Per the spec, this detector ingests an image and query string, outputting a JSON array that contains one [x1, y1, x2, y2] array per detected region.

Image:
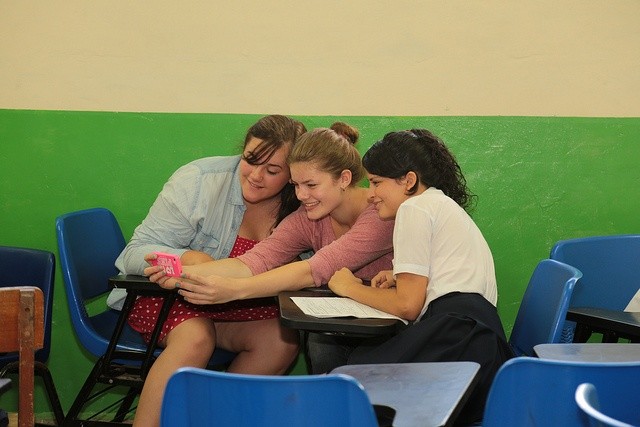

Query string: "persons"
[[103, 115, 309, 425], [142, 121, 393, 309], [326, 124, 514, 423]]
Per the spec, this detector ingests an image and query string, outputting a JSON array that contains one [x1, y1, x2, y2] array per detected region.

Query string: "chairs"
[[482, 357, 640, 427], [550, 235, 639, 342], [575, 383, 633, 426], [467, 258, 583, 427], [0, 286, 45, 427], [55, 207, 237, 427], [0, 246, 65, 426], [152, 366, 379, 427]]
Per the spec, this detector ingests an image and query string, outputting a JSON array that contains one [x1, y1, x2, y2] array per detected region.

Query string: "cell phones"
[[150, 252, 182, 280]]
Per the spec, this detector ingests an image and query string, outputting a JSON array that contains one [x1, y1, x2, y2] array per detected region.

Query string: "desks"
[[325, 361, 482, 427], [566, 307, 640, 343], [277, 289, 402, 337], [533, 343, 640, 364]]
